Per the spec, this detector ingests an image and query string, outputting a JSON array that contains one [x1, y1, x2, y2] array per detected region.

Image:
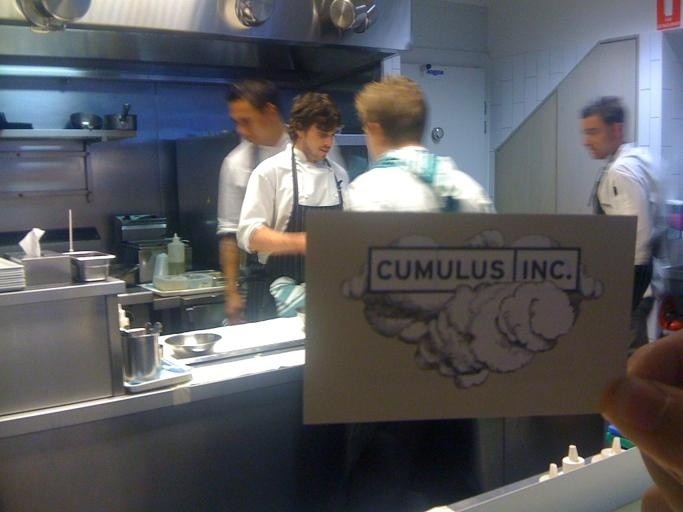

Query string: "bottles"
[[167, 233, 185, 275]]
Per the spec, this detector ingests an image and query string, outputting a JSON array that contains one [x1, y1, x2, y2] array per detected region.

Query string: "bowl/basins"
[[165, 333, 222, 357]]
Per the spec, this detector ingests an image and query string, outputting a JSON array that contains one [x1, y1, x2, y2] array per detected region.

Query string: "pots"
[[105, 103, 137, 130]]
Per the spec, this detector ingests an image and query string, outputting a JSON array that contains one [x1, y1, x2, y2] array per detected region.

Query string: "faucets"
[[120, 264, 140, 278]]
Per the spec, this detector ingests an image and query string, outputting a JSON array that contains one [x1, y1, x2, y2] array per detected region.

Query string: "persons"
[[334, 75, 496, 511], [596, 329, 682, 512], [577, 92, 666, 361], [214, 75, 292, 327], [236, 89, 349, 321]]
